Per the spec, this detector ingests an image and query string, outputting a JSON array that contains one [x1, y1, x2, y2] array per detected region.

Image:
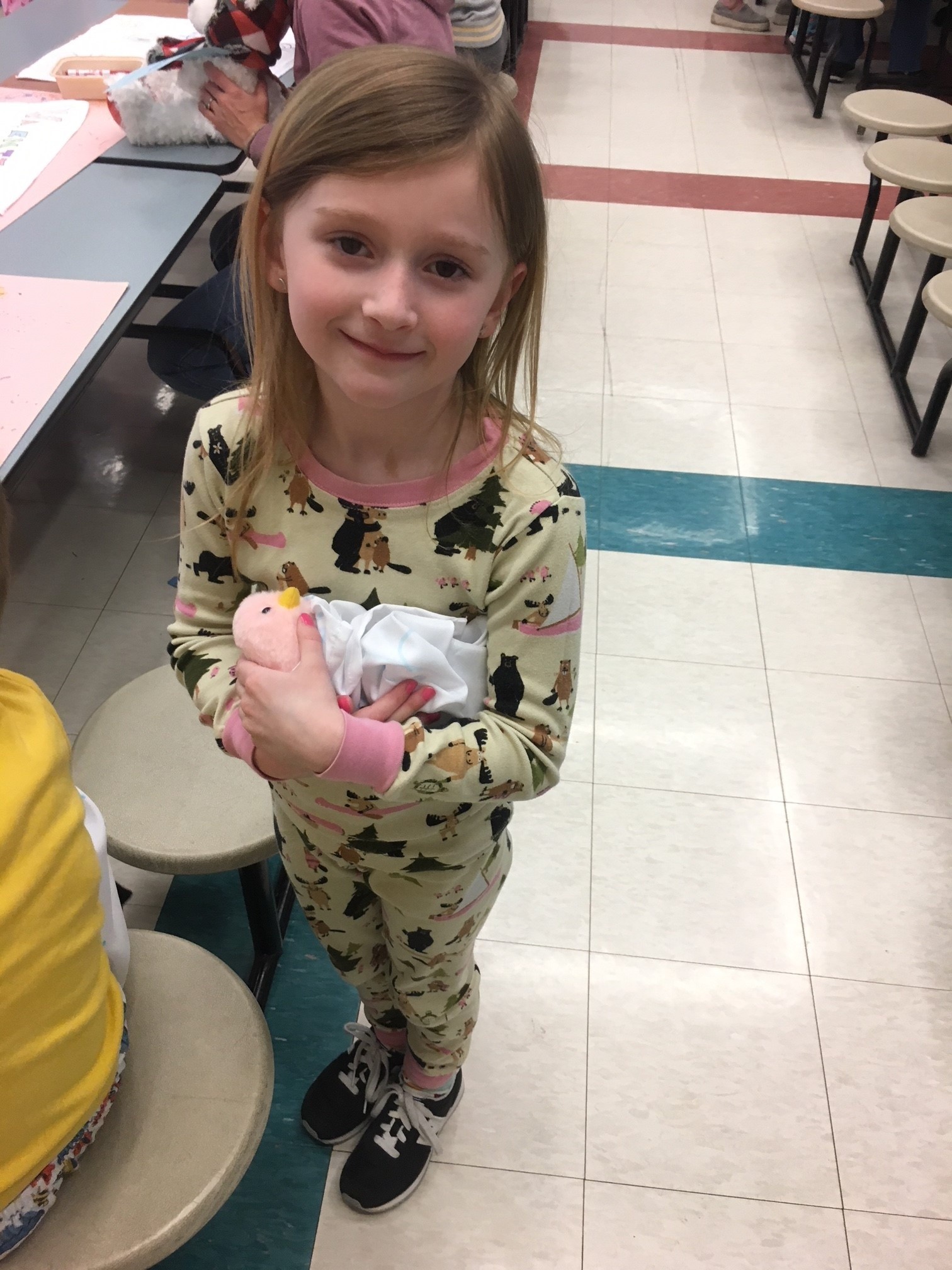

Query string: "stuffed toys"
[[106, 0, 296, 147], [232, 586, 486, 721]]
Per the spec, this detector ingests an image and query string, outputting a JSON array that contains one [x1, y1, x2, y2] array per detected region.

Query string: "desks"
[[0, 0, 248, 488]]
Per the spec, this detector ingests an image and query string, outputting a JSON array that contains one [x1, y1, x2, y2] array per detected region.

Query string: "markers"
[[64, 67, 133, 76]]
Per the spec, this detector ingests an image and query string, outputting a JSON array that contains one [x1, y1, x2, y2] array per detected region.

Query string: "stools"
[[785, 0, 952, 458], [0, 664, 302, 1270]]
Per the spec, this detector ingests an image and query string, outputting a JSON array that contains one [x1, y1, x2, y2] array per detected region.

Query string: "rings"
[[207, 97, 214, 109]]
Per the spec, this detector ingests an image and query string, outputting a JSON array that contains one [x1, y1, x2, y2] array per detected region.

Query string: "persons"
[[1, 670, 131, 1264], [165, 50, 587, 1213], [449, 0, 507, 79], [146, 0, 457, 400], [711, 0, 931, 90]]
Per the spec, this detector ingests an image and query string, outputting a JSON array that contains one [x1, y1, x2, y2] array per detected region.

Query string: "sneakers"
[[772, 0, 803, 26], [711, 0, 771, 32], [829, 60, 857, 84], [300, 1022, 404, 1145], [339, 1068, 465, 1214], [789, 22, 832, 57]]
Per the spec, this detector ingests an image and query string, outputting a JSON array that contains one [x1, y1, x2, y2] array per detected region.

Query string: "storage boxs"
[[51, 56, 144, 99]]
